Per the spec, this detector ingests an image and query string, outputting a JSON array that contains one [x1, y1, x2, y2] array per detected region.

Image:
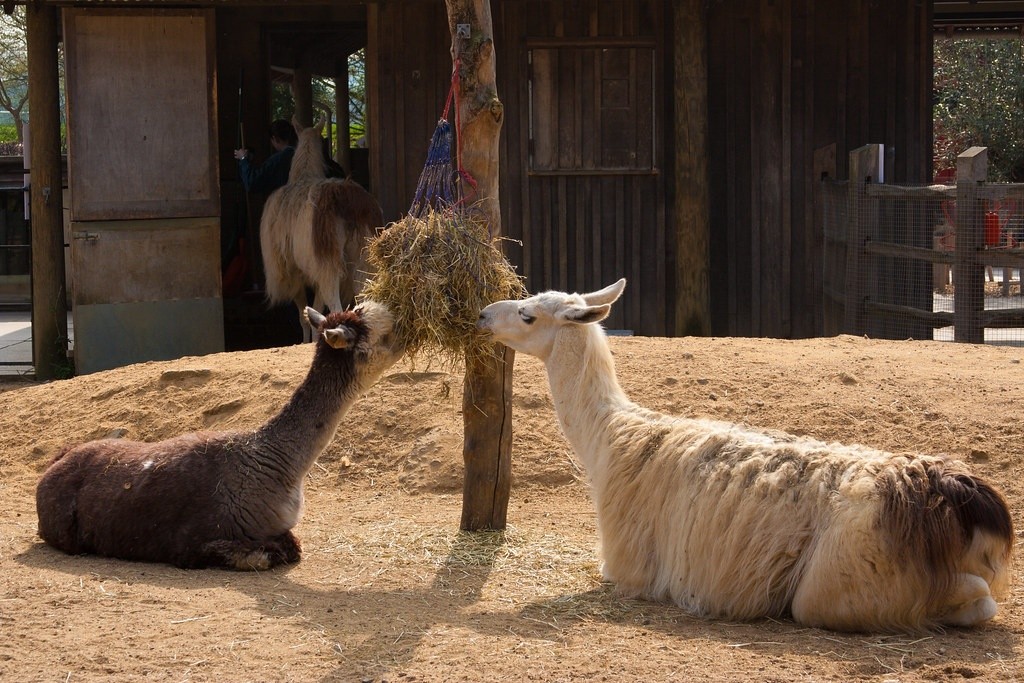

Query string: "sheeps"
[[259, 112, 387, 345], [479, 278, 1015, 639], [34, 299, 407, 575]]
